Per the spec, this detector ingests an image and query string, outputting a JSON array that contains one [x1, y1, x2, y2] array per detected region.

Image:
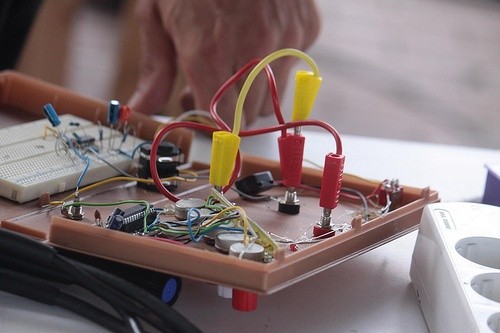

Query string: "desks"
[[0, 108, 500, 333]]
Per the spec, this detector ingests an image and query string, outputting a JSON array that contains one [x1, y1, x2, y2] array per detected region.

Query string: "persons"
[[0, 0, 322, 137]]
